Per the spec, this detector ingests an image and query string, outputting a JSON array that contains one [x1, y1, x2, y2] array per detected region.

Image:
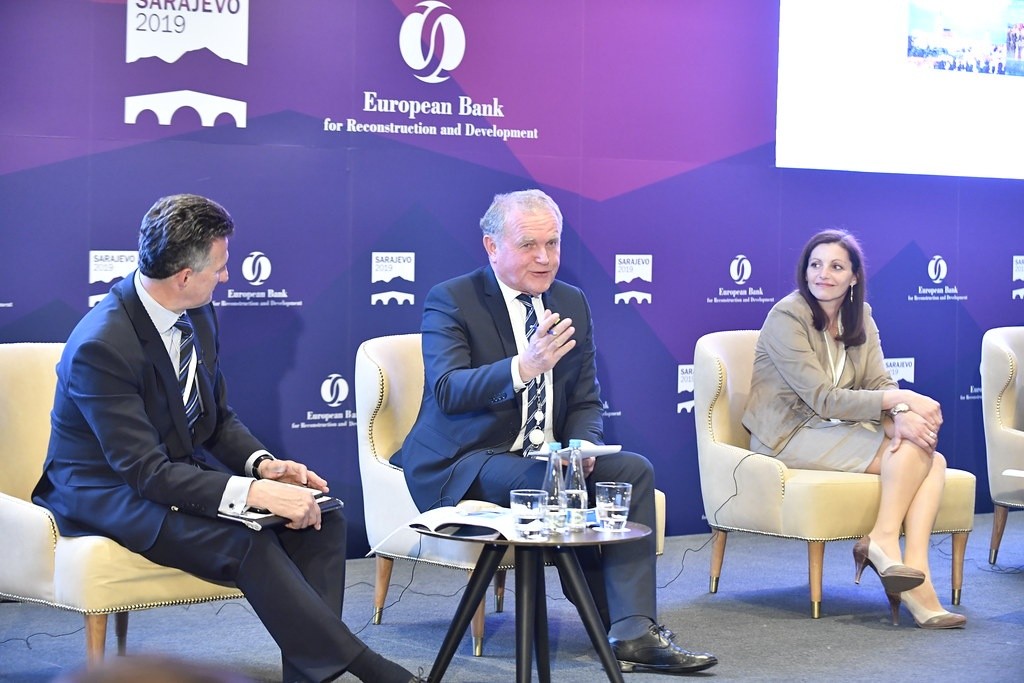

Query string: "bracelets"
[[251, 454, 274, 480]]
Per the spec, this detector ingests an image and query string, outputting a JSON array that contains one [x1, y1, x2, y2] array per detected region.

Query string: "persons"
[[30, 192, 429, 683], [388, 188, 718, 674], [741, 228, 968, 631]]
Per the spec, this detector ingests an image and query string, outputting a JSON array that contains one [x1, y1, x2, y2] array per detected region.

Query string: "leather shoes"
[[605, 623, 718, 672]]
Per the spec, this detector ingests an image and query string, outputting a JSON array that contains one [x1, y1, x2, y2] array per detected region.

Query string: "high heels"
[[852, 534, 926, 593], [885, 588, 967, 630]]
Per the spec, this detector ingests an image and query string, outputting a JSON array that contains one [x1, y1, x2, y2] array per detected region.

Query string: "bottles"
[[537, 441, 566, 505], [565, 439, 587, 491]]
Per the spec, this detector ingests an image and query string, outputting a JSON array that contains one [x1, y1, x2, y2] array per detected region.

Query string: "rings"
[[929, 432, 935, 438]]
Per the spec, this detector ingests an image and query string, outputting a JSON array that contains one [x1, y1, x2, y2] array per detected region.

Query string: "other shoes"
[[410, 667, 431, 683]]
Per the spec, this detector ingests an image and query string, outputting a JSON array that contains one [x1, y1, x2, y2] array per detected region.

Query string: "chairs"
[[354, 329, 666, 655], [978, 323, 1024, 567], [695, 328, 977, 620], [0, 343, 244, 683]]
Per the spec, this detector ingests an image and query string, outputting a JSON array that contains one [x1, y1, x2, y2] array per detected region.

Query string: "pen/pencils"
[[530, 325, 557, 336]]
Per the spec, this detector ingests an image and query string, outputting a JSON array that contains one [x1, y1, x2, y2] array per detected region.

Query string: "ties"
[[516, 292, 546, 459], [174, 314, 204, 472]]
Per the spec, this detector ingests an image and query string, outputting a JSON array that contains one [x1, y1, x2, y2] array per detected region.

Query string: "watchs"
[[890, 403, 911, 416]]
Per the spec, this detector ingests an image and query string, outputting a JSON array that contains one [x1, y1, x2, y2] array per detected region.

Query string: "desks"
[[400, 513, 657, 683]]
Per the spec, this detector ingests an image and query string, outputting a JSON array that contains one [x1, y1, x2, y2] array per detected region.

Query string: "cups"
[[558, 488, 588, 533], [510, 489, 549, 540], [540, 505, 565, 539], [596, 483, 632, 531]]
